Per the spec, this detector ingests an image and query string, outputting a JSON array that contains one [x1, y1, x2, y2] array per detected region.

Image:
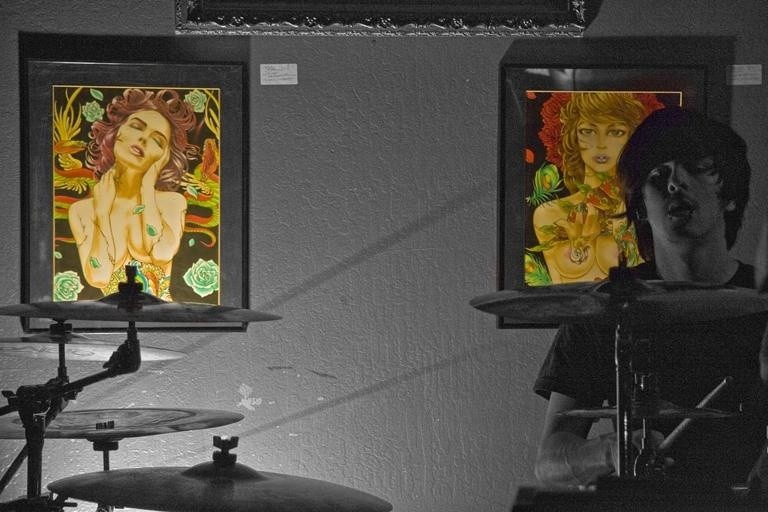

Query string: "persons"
[[68, 88, 200, 302], [532, 107, 768, 483], [533, 92, 646, 284]]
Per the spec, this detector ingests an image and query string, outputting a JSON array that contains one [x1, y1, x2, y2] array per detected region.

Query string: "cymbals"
[[48, 461, 392, 512], [469, 280, 767, 327], [0, 407, 244, 438], [0, 300, 283, 321], [557, 399, 738, 421], [0, 331, 188, 361]]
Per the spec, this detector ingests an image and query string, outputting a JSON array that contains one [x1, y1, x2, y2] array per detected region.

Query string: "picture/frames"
[[496, 60, 708, 331], [18, 56, 252, 333]]
